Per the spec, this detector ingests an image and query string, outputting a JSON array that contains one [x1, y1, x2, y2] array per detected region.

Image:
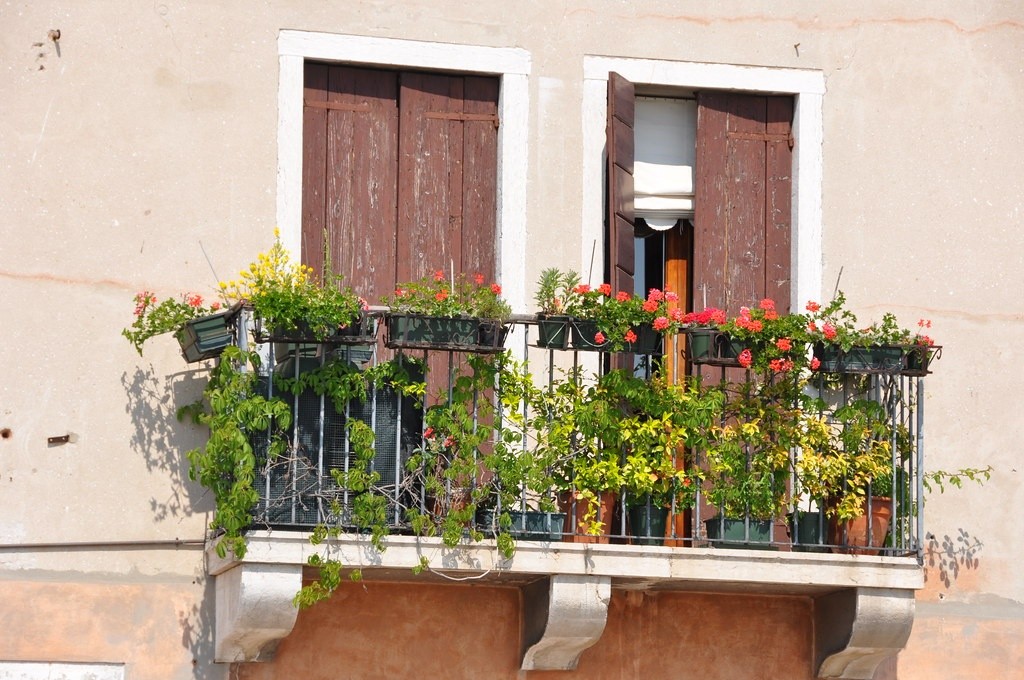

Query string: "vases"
[[786, 511, 831, 553], [272, 319, 337, 340], [702, 516, 771, 550], [902, 350, 933, 377], [475, 508, 568, 541], [536, 312, 658, 356], [338, 312, 361, 337], [274, 317, 376, 363], [628, 503, 671, 547], [389, 314, 480, 347], [687, 332, 765, 369], [173, 312, 233, 363], [481, 323, 509, 348], [827, 495, 893, 556], [811, 341, 904, 376]]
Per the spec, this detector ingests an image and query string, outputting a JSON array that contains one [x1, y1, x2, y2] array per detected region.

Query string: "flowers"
[[117, 223, 998, 616]]
